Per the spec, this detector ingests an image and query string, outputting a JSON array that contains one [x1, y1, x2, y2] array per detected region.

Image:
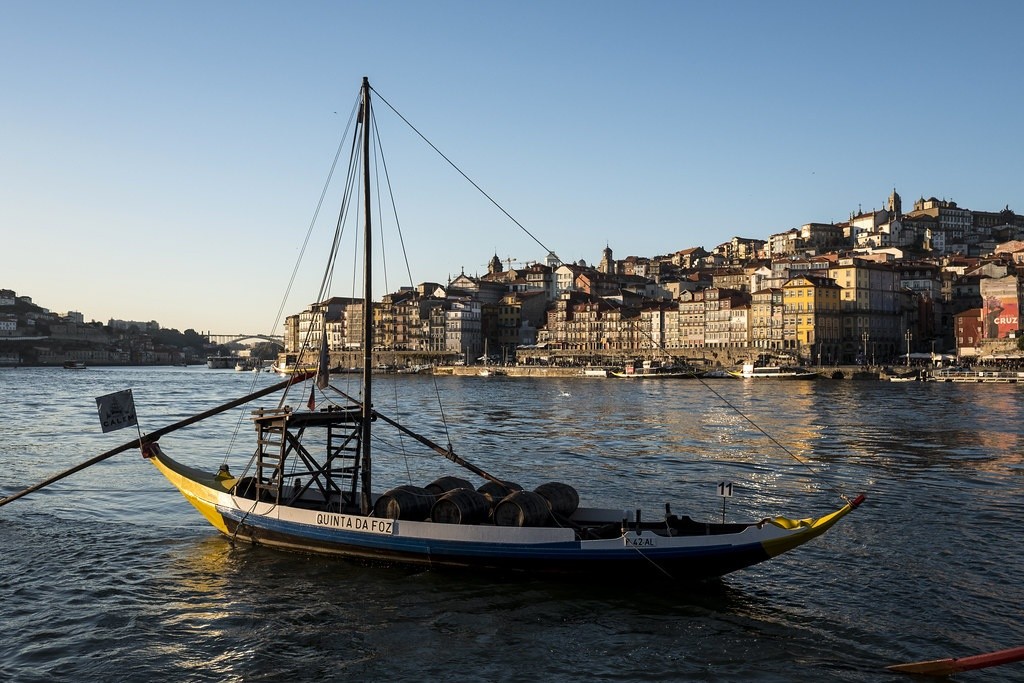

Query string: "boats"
[[889, 376, 918, 381], [608, 357, 705, 378], [725, 352, 826, 379]]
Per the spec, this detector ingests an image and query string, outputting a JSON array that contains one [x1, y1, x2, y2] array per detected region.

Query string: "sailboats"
[[97, 78, 872, 578], [475, 337, 496, 376]]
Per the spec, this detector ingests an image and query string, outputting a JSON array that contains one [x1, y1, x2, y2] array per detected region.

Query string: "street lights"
[[861, 331, 870, 363], [904, 329, 913, 366]]
